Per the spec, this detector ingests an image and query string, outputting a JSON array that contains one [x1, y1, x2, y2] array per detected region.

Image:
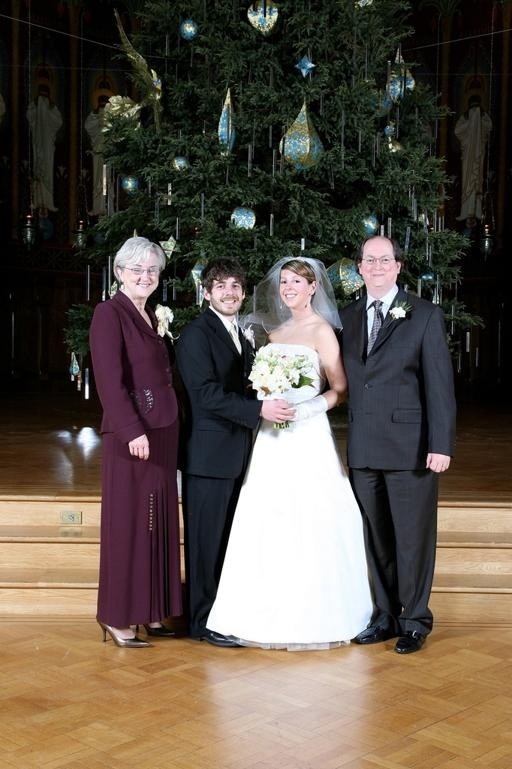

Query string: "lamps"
[[71, 186, 89, 251], [19, 177, 39, 252], [479, 191, 497, 262]]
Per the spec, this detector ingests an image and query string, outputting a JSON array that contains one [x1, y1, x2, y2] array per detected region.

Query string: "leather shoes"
[[190, 632, 242, 647], [395, 630, 427, 653], [355, 624, 394, 644]]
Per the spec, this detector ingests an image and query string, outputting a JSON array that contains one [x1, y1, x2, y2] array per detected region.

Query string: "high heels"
[[136, 622, 176, 637], [97, 619, 151, 648]]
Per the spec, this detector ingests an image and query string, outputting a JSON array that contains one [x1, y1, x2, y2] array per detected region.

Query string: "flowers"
[[155, 306, 181, 340], [389, 300, 413, 320], [244, 323, 256, 349], [247, 345, 316, 429]]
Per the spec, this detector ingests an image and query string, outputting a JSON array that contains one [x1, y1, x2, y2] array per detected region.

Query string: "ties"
[[231, 323, 244, 355], [367, 300, 384, 356]]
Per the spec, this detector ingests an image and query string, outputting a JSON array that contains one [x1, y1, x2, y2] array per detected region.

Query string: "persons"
[[88, 237, 183, 647], [172, 258, 296, 647], [205, 255, 374, 652], [330, 235, 458, 653]]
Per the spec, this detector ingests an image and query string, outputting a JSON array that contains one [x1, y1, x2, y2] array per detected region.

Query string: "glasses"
[[121, 266, 160, 275], [361, 256, 395, 265]]
